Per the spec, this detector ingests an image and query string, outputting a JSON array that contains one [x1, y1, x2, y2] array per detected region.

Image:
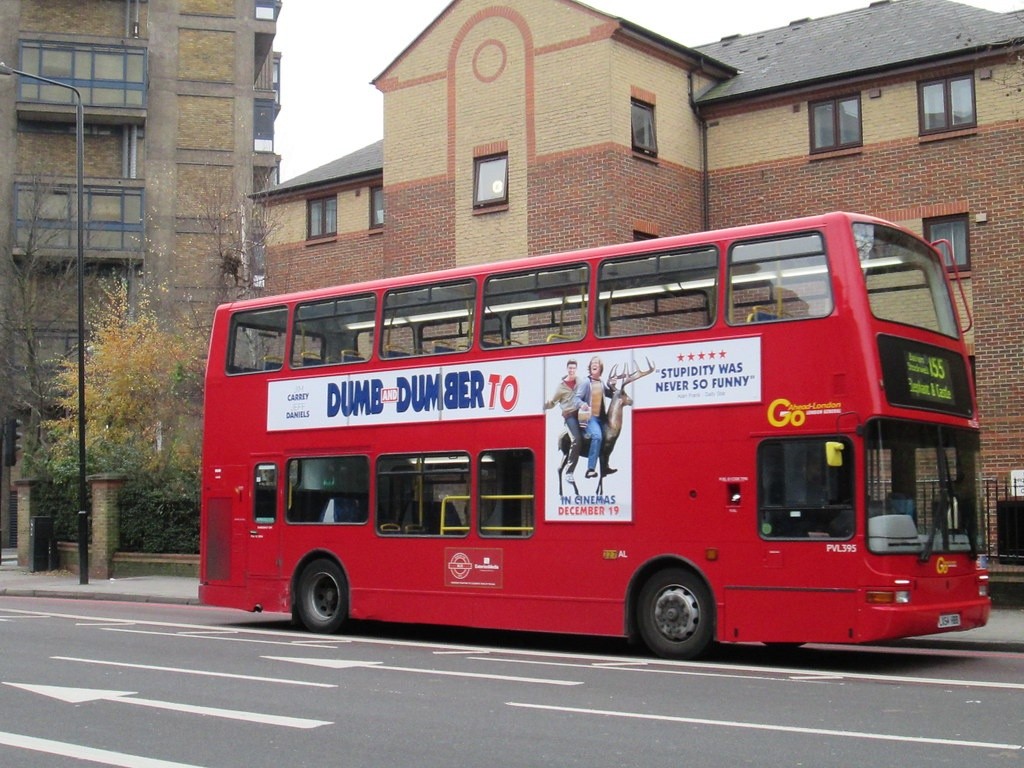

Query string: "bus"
[[198, 210, 992, 662]]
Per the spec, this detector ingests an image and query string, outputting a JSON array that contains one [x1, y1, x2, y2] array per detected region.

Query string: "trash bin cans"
[[29, 516, 52, 572]]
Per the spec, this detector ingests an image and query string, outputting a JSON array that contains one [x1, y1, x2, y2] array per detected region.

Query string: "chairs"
[[262, 334, 574, 370], [406, 524, 426, 534], [746, 307, 793, 323], [380, 524, 402, 534]]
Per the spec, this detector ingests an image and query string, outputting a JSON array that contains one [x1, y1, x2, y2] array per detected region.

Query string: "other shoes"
[[566, 474, 573, 482], [585, 470, 597, 478], [606, 467, 618, 475]]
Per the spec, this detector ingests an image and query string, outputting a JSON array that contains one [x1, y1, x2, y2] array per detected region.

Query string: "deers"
[[557, 356, 656, 498]]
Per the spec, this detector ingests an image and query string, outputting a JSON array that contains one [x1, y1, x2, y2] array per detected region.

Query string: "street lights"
[[0, 62, 88, 587]]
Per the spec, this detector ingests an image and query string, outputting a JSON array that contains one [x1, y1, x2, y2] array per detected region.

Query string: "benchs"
[[319, 497, 369, 523]]
